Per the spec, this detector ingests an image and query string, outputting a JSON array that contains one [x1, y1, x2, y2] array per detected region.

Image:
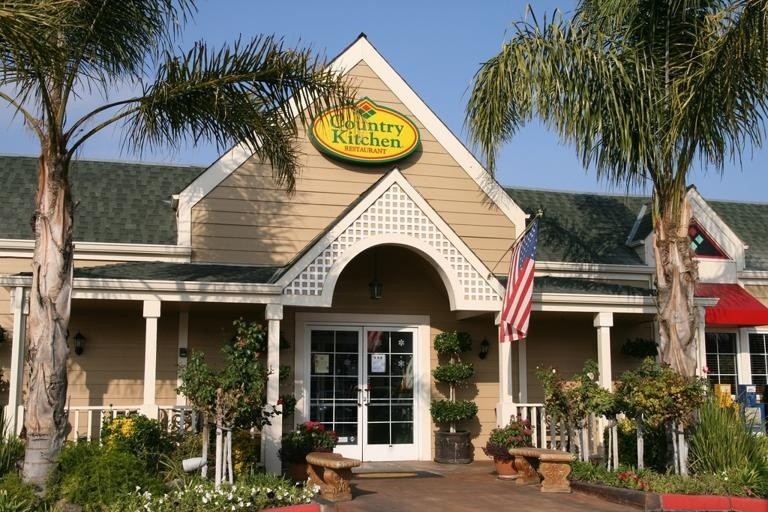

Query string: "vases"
[[281, 448, 333, 481], [493, 453, 519, 475]]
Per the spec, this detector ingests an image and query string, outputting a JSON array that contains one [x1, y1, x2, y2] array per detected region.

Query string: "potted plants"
[[430, 328, 478, 464]]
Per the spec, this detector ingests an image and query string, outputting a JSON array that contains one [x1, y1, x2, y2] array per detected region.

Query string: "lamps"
[[73, 330, 87, 356], [479, 337, 490, 359], [369, 246, 385, 300]]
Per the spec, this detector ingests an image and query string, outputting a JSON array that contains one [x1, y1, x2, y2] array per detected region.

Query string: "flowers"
[[281, 420, 338, 451], [481, 414, 536, 457]]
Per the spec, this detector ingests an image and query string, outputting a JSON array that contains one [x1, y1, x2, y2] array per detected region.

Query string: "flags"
[[498, 217, 538, 344]]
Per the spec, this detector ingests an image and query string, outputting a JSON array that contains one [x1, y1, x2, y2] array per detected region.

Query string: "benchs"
[[304, 450, 361, 501], [508, 447, 576, 494]]
